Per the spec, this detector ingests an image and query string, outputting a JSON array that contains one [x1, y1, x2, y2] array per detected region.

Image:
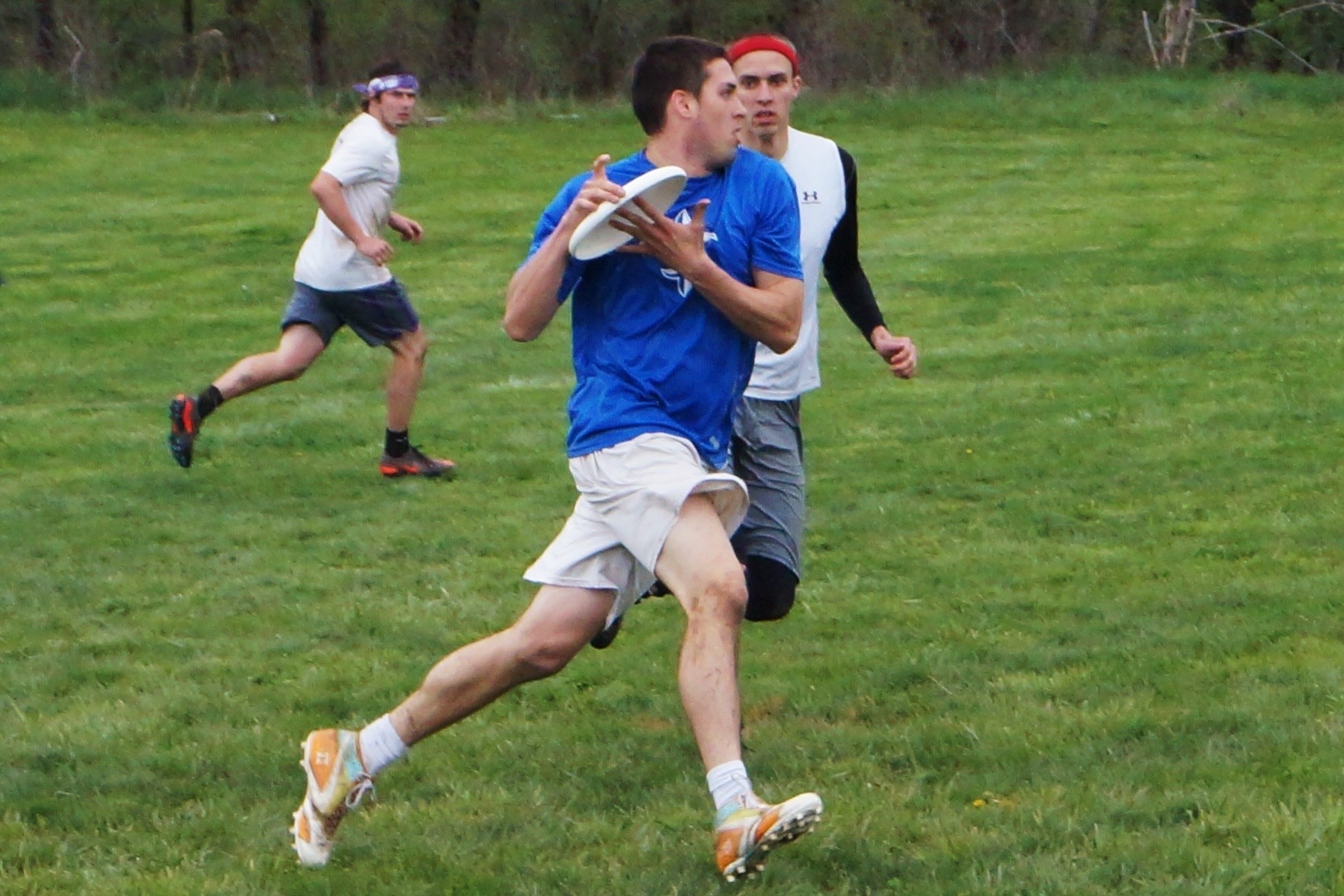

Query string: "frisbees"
[[567, 165, 689, 262]]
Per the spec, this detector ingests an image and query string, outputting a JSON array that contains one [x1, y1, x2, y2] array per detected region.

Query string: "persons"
[[287, 37, 825, 883], [168, 61, 455, 475], [590, 31, 917, 650]]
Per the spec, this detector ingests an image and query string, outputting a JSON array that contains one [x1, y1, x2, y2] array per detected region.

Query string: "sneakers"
[[590, 614, 623, 649], [294, 729, 364, 867], [382, 449, 453, 477], [717, 792, 824, 883], [169, 392, 199, 468]]
